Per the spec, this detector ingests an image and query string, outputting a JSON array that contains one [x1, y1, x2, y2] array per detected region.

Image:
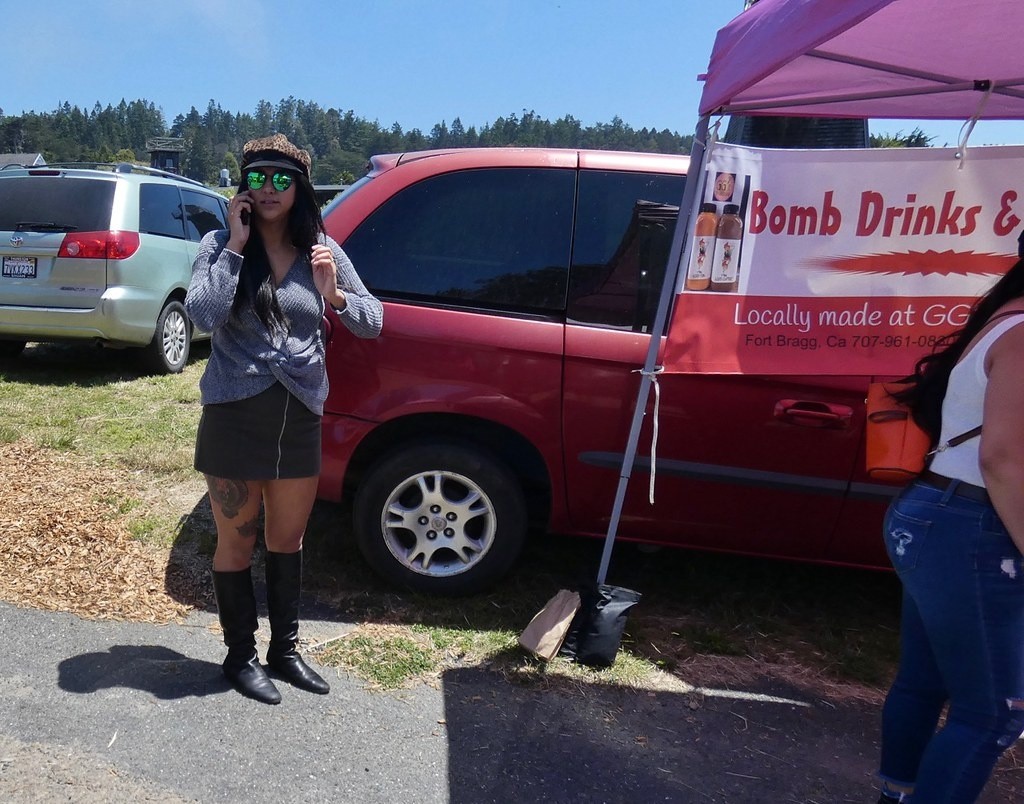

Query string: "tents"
[[592, 0, 1023, 593]]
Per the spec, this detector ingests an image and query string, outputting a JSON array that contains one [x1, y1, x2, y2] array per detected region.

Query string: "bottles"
[[686, 203, 720, 291], [711, 205, 743, 292]]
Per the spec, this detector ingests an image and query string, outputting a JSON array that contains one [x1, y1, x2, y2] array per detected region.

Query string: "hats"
[[243, 132, 311, 181]]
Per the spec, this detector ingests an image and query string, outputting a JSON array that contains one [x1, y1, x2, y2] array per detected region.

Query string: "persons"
[[184, 135, 384, 704], [877, 230, 1023, 804]]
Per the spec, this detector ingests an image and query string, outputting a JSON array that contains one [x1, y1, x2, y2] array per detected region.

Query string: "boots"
[[210, 565, 282, 704], [265, 549, 330, 694]]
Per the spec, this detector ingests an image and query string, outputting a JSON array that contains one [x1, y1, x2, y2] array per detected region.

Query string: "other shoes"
[[877, 792, 907, 804]]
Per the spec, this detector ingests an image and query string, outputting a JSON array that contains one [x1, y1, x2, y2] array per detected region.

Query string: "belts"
[[918, 469, 991, 502]]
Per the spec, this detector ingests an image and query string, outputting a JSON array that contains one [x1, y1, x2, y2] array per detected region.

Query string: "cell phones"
[[239, 184, 248, 224]]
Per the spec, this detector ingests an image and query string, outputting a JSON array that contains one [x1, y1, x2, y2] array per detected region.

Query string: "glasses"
[[246, 169, 296, 193]]
